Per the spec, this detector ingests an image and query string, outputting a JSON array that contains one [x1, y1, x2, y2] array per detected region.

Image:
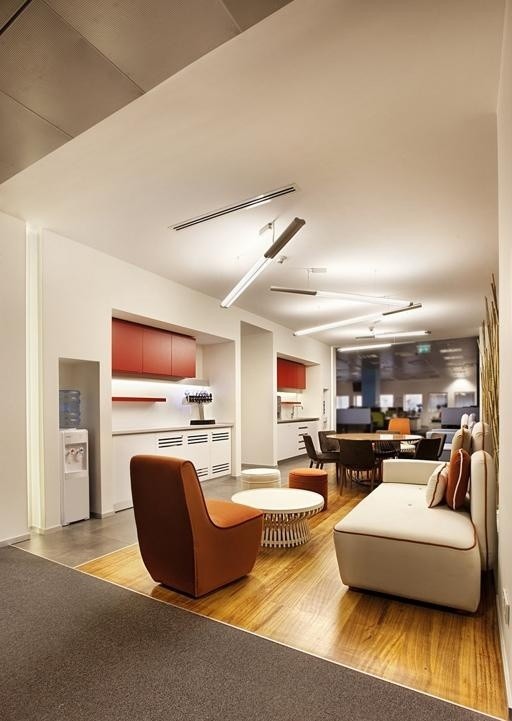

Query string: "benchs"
[[441, 407, 479, 427], [337, 408, 373, 432]]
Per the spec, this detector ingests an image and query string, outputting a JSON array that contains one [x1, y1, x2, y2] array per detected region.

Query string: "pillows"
[[424, 426, 471, 511]]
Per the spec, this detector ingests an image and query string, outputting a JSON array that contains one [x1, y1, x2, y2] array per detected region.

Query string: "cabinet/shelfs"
[[278, 422, 320, 460], [156, 427, 233, 483], [277, 358, 306, 390], [113, 318, 197, 380]]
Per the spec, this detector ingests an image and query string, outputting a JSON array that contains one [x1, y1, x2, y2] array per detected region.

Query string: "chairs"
[[303, 418, 447, 496], [130, 454, 264, 598]]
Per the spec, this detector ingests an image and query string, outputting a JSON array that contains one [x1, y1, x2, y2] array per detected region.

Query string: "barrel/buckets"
[[60, 389, 81, 428]]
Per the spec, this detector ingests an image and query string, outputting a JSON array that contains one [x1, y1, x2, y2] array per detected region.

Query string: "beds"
[[333, 460, 497, 614]]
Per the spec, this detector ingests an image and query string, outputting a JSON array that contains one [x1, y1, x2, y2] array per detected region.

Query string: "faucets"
[[292, 405, 303, 414]]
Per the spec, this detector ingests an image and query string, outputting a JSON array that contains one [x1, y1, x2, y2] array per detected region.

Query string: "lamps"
[[219, 217, 432, 354]]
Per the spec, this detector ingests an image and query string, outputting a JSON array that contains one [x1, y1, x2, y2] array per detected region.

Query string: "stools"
[[241, 468, 281, 488], [289, 468, 328, 511]]
